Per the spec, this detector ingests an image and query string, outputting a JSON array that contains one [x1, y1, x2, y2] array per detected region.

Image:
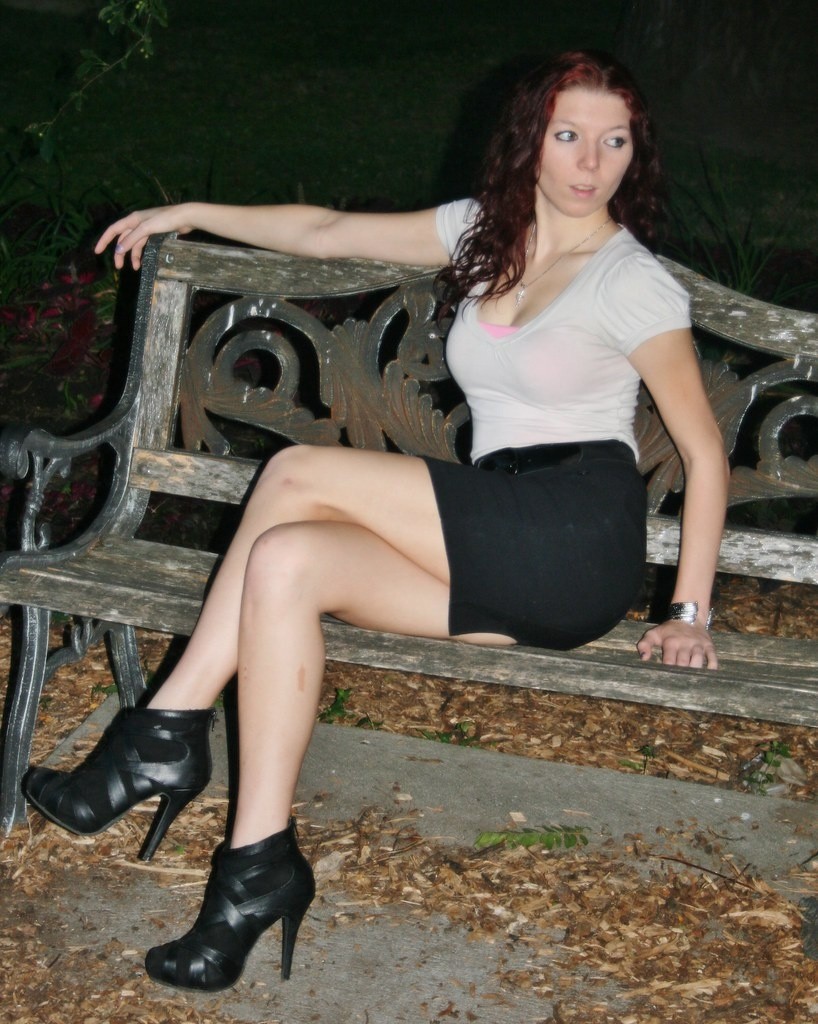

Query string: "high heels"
[[23, 706, 217, 862], [144, 817, 315, 993]]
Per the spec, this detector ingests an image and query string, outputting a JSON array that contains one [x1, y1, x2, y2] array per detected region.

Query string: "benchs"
[[0, 233, 817, 855]]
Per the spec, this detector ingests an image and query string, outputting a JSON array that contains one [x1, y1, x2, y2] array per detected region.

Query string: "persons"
[[19, 43, 731, 993]]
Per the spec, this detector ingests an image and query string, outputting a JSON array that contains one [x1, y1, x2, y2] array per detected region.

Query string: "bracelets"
[[665, 602, 714, 630]]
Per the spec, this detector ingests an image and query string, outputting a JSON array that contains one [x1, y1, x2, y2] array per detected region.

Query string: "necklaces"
[[515, 217, 613, 306]]
